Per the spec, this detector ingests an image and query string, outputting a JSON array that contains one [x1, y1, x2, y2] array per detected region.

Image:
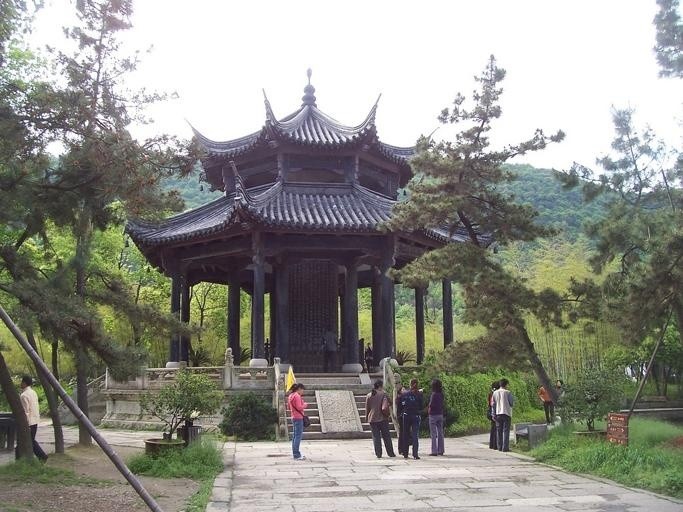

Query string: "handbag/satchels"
[[300, 415, 312, 428], [382, 397, 391, 416], [424, 415, 447, 426], [486, 410, 496, 424]]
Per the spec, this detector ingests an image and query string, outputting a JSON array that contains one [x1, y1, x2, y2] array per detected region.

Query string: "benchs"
[[510, 421, 550, 451]]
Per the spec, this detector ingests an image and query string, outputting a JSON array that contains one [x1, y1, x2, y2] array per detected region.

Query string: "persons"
[[538, 386, 556, 427], [364, 379, 396, 457], [287, 382, 306, 461], [320, 324, 373, 373], [488, 381, 500, 451], [491, 377, 514, 452], [555, 379, 567, 403], [398, 378, 424, 459], [14, 375, 48, 464], [394, 386, 413, 455], [428, 378, 446, 457]]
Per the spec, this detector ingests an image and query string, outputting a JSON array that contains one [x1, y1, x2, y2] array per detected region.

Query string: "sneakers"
[[293, 455, 307, 462], [375, 451, 446, 460], [489, 445, 513, 454]]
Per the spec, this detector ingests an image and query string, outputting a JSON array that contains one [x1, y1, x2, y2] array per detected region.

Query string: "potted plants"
[[566, 366, 636, 439], [137, 368, 229, 465]]
